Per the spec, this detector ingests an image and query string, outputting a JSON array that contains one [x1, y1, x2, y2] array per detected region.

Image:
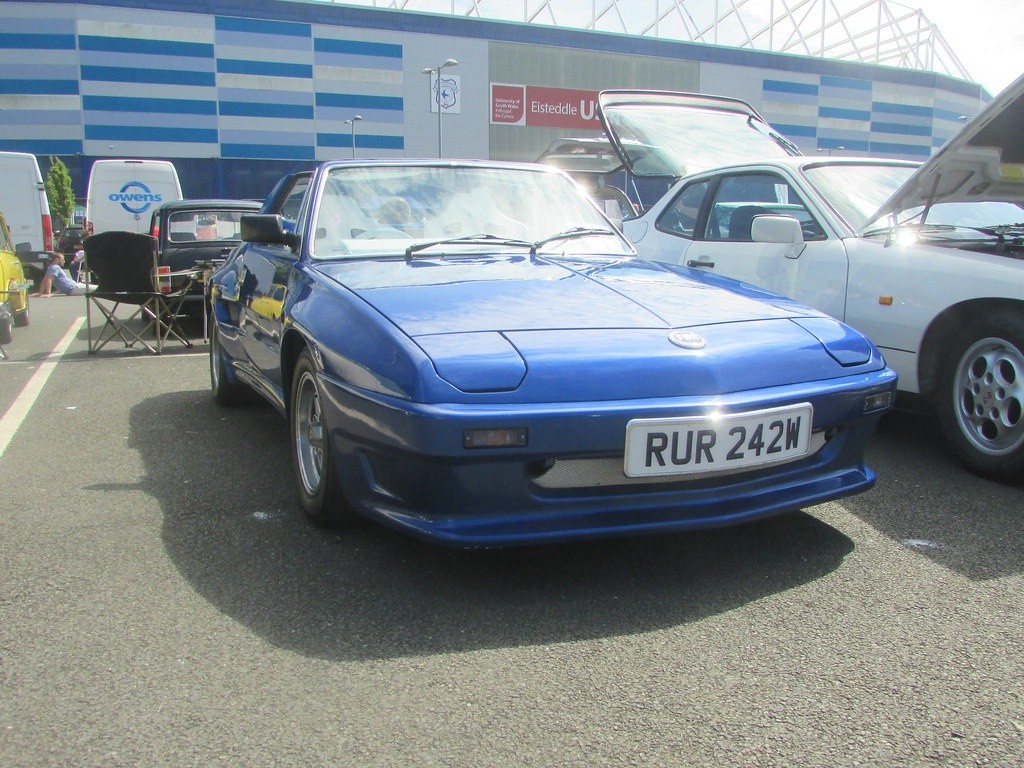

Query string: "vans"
[[0, 151, 53, 291], [83, 160, 184, 236]]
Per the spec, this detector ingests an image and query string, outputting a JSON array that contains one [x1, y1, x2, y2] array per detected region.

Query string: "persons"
[[33, 252, 98, 301], [671, 177, 709, 226], [69, 250, 85, 282], [376, 197, 423, 238], [623, 204, 639, 220], [195, 219, 218, 240]]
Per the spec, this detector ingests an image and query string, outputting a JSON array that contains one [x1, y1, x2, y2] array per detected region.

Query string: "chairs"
[[172, 231, 197, 241], [83, 231, 199, 355], [728, 205, 772, 240]]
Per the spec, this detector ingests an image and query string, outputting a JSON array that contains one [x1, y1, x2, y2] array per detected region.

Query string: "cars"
[[0, 212, 30, 344], [532, 138, 662, 237], [54, 224, 86, 259], [589, 71, 1024, 478], [207, 159, 899, 551]]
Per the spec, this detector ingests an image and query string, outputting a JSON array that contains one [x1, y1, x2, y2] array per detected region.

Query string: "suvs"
[[139, 198, 294, 322]]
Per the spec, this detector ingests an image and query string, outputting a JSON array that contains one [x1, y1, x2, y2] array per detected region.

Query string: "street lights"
[[421, 59, 459, 159], [344, 115, 362, 159]]
